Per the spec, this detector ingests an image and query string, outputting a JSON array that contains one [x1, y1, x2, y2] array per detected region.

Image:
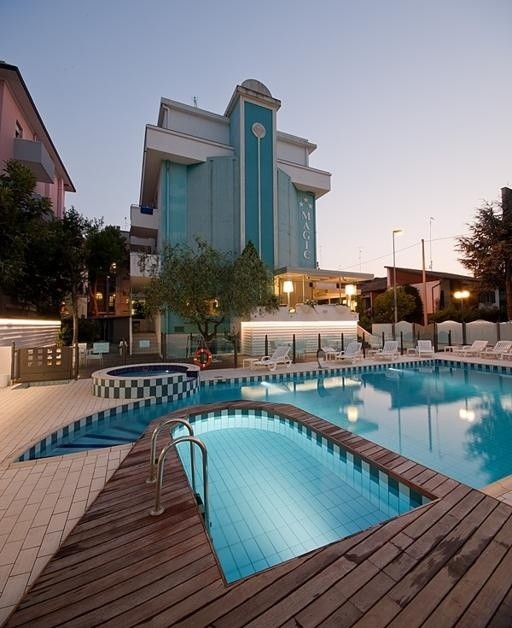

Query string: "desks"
[[324, 352, 339, 362], [243, 358, 259, 369]]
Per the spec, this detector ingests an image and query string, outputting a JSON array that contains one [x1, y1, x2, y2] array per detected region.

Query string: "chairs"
[[252, 345, 293, 372], [334, 339, 512, 364]]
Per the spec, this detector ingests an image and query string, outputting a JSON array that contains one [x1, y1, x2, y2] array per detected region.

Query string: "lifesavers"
[[193, 349, 212, 368]]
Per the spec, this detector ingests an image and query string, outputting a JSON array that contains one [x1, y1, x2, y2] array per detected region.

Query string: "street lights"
[[281, 278, 294, 306], [344, 387, 359, 425], [344, 282, 359, 309], [456, 396, 475, 422], [453, 289, 473, 322], [392, 227, 405, 321]]
